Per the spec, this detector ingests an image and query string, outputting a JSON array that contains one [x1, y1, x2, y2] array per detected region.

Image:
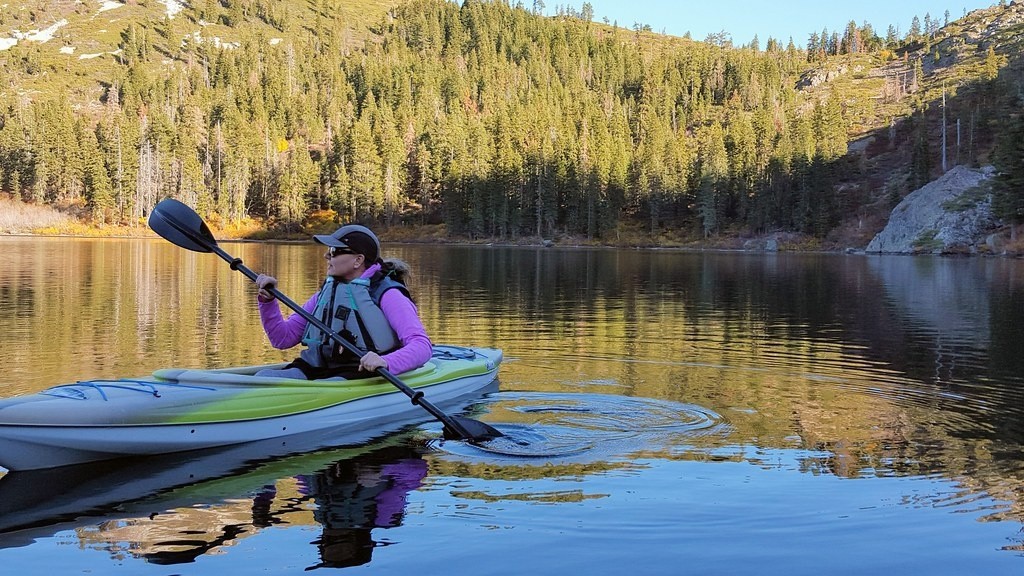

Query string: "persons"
[[251, 446, 429, 571], [255, 224, 433, 382]]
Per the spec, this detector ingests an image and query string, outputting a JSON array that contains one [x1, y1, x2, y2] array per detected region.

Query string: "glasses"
[[328, 246, 359, 258]]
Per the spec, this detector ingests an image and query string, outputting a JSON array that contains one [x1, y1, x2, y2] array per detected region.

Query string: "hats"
[[312, 224, 380, 263]]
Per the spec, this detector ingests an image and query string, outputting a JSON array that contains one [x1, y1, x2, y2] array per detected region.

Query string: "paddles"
[[149, 197, 499, 444]]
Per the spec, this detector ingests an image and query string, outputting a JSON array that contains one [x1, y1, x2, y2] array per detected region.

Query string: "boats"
[[0, 340, 507, 472]]
